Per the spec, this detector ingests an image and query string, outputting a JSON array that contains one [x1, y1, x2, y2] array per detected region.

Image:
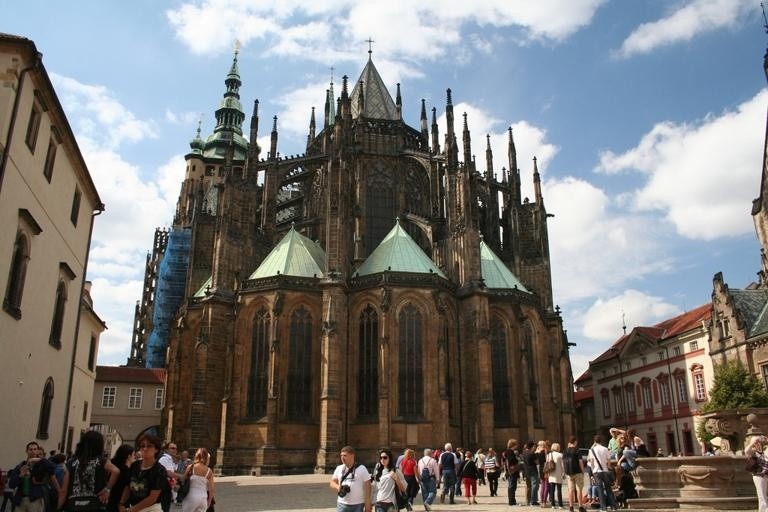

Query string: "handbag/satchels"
[[161, 480, 171, 512], [397, 493, 408, 509], [177, 475, 189, 502], [745, 455, 768, 475], [543, 460, 555, 473], [598, 472, 615, 483]]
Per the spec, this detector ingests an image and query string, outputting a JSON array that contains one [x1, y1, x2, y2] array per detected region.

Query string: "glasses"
[[380, 456, 387, 459]]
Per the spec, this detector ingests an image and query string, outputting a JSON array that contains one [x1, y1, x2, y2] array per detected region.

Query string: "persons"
[[678, 450, 684, 458], [701, 449, 713, 456], [654, 448, 666, 459], [667, 452, 675, 458], [330, 426, 650, 511], [745, 435, 768, 512], [0, 427, 217, 512]]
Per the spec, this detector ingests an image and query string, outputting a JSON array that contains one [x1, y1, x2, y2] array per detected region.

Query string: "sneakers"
[[424, 502, 431, 511], [407, 502, 412, 511], [449, 501, 456, 504], [600, 506, 616, 512], [441, 493, 445, 502], [491, 490, 497, 496], [527, 500, 595, 512], [468, 501, 477, 504]]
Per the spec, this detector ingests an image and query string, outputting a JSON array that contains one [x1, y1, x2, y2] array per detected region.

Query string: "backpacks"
[[564, 448, 580, 475], [421, 458, 432, 482]]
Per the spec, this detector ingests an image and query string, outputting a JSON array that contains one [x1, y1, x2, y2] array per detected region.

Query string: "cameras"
[[338, 486, 350, 498]]
